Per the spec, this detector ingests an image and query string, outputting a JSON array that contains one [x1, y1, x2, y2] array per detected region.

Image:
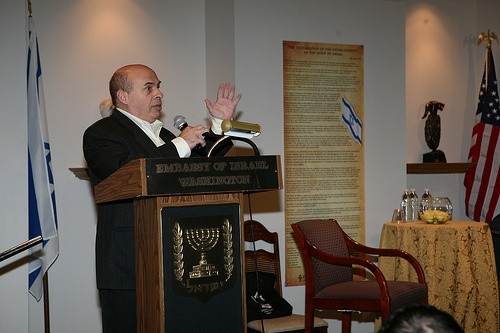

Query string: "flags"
[[26, 14, 60, 301], [463, 47, 500, 224]]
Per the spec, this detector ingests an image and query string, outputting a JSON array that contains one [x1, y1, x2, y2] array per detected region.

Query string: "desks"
[[378, 221, 499, 333]]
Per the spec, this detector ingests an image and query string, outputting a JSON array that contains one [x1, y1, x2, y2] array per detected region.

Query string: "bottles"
[[410, 189, 419, 222], [401, 189, 412, 222], [422, 188, 432, 199]]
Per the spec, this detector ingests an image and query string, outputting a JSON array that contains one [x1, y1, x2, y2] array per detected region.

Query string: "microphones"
[[173, 115, 207, 157]]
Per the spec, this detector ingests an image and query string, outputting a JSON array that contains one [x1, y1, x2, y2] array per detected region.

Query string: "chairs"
[[290, 218, 428, 333], [245, 221, 329, 333]]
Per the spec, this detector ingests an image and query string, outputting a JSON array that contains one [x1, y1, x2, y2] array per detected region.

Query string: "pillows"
[[246, 272, 294, 322]]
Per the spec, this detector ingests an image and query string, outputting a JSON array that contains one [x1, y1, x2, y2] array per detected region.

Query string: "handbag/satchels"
[[247, 272, 293, 323]]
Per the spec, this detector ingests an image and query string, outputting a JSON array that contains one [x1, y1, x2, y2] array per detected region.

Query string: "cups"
[[392, 208, 403, 224], [419, 196, 452, 223]]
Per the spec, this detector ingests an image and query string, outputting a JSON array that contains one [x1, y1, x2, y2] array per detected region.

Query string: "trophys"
[[186, 228, 221, 278]]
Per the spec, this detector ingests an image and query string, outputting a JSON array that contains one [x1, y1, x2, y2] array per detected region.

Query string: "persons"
[[83, 65, 242, 333]]
